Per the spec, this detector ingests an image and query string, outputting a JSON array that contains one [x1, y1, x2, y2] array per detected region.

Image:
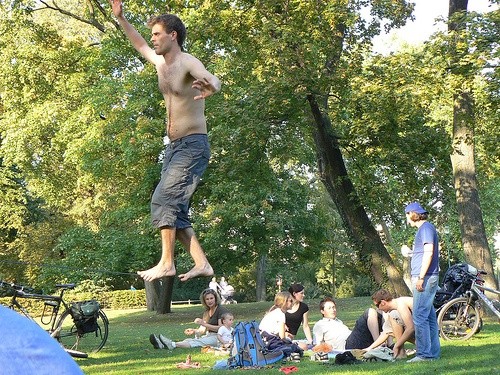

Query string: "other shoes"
[[407, 357, 426, 362], [404, 348, 416, 355]]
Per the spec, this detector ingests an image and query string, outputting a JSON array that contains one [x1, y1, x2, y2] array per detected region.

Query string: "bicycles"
[[436, 269, 500, 343], [0, 280, 110, 357]]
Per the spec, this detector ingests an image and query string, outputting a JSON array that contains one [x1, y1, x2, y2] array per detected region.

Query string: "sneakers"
[[309, 352, 329, 363], [150, 333, 163, 350], [159, 334, 174, 350], [343, 351, 362, 363], [335, 354, 362, 364], [282, 352, 300, 364]]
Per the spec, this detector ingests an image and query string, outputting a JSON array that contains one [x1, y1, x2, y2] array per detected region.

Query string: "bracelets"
[[418, 277, 424, 280]]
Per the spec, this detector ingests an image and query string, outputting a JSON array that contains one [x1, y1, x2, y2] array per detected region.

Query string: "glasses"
[[287, 299, 293, 303], [375, 299, 384, 308]]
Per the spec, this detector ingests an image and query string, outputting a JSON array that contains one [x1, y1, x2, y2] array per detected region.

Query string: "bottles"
[[194, 330, 200, 339], [186, 355, 190, 365]]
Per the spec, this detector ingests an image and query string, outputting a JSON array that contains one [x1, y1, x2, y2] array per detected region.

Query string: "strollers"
[[432, 263, 488, 320], [217, 288, 237, 305]]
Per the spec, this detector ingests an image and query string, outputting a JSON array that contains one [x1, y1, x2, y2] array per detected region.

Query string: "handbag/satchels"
[[71, 301, 99, 334]]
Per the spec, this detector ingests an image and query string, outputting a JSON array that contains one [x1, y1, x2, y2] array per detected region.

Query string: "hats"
[[405, 203, 427, 214]]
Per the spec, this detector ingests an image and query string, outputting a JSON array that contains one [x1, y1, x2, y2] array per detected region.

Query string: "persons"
[[271, 284, 312, 346], [0, 305, 86, 375], [362, 289, 416, 359], [209, 277, 223, 301], [150, 288, 228, 350], [217, 312, 234, 347], [107, 0, 221, 281], [312, 299, 396, 353], [401, 203, 442, 362], [219, 276, 227, 290], [258, 292, 293, 344]]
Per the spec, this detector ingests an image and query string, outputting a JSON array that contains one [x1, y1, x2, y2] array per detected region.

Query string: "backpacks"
[[227, 320, 266, 370]]
